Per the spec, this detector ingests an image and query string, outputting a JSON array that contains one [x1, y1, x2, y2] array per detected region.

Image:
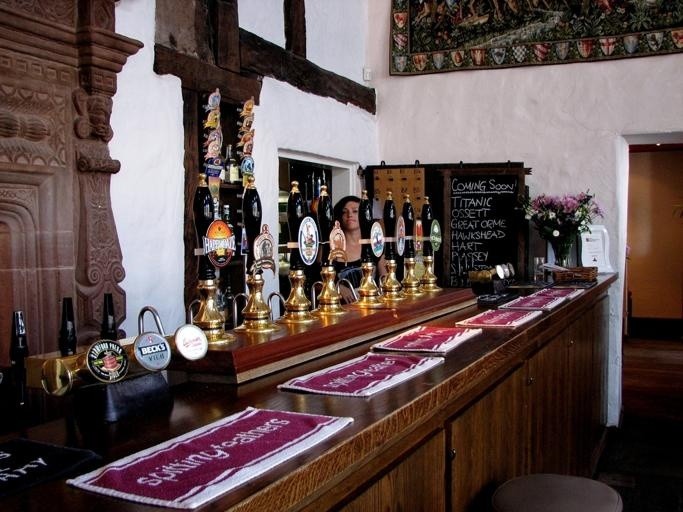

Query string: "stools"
[[489, 473, 623, 511]]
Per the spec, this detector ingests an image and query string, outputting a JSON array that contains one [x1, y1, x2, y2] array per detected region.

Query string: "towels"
[[497, 288, 583, 309], [371, 325, 482, 353], [66, 403, 356, 509], [454, 309, 545, 330], [274, 351, 447, 397]]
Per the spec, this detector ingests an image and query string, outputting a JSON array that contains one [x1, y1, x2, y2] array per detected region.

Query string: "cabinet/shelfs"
[[184, 82, 250, 332]]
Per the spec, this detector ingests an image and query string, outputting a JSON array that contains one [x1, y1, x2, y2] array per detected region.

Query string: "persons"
[[329, 195, 364, 305]]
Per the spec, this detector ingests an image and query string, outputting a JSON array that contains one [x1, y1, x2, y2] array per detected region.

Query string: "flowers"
[[515, 186, 605, 267]]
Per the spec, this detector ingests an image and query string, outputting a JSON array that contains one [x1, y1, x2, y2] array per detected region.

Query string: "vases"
[[552, 243, 574, 270]]
